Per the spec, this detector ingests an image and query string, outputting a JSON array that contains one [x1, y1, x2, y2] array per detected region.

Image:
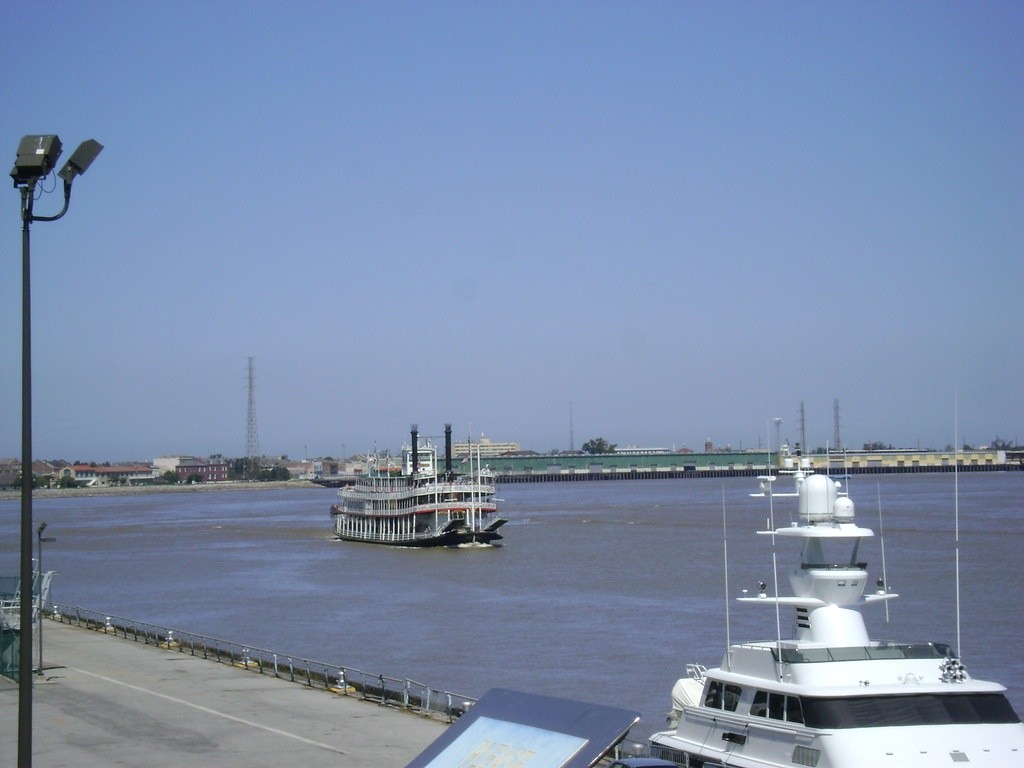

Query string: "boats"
[[638, 418, 1022, 768], [327, 423, 509, 544]]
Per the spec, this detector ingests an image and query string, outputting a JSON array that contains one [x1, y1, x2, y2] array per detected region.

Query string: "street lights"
[[32, 523, 49, 677], [7, 134, 104, 754]]
